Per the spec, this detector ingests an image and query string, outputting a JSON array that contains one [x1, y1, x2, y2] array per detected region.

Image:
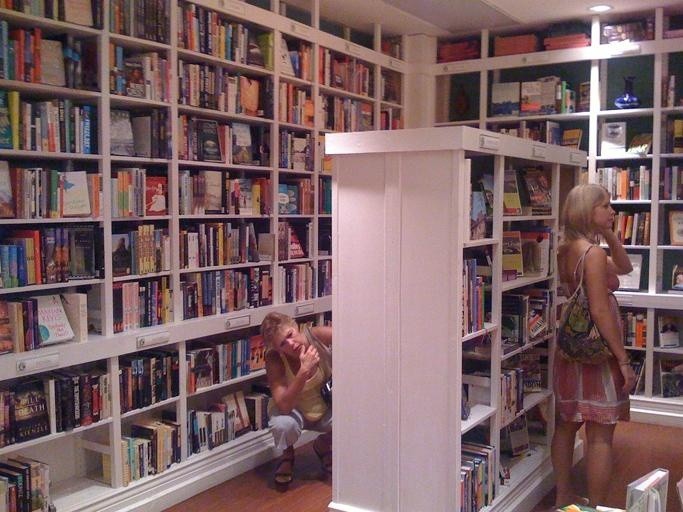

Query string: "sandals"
[[274, 457, 296, 486], [313, 434, 332, 474]]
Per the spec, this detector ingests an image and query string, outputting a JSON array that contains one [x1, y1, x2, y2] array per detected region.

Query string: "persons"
[[548, 181, 639, 508], [260, 310, 333, 485]]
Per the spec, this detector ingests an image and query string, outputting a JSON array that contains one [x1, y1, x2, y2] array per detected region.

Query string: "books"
[[459, 162, 557, 512], [278, 14, 408, 326], [85, 0, 180, 488], [176, 0, 271, 456], [1, 0, 112, 512], [437, 13, 683, 398]]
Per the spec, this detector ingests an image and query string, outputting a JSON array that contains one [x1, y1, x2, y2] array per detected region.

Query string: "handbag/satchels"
[[555, 277, 625, 367]]
